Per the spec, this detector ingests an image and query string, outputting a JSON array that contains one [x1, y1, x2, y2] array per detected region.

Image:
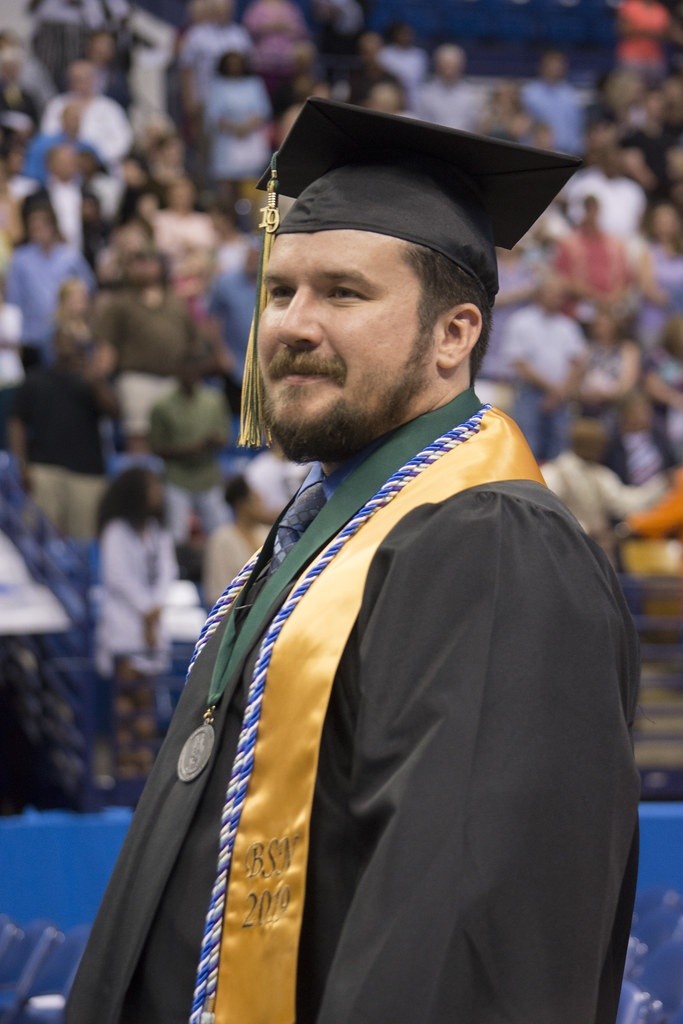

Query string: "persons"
[[66, 98, 649, 1024], [2, 0, 680, 787]]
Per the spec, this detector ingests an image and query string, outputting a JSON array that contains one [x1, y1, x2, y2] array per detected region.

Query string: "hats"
[[238, 93, 591, 446]]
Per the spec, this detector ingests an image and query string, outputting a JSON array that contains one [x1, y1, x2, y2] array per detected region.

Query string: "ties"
[[265, 482, 330, 583]]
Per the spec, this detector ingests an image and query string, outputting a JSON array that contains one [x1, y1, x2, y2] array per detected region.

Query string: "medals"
[[176, 718, 217, 784]]
